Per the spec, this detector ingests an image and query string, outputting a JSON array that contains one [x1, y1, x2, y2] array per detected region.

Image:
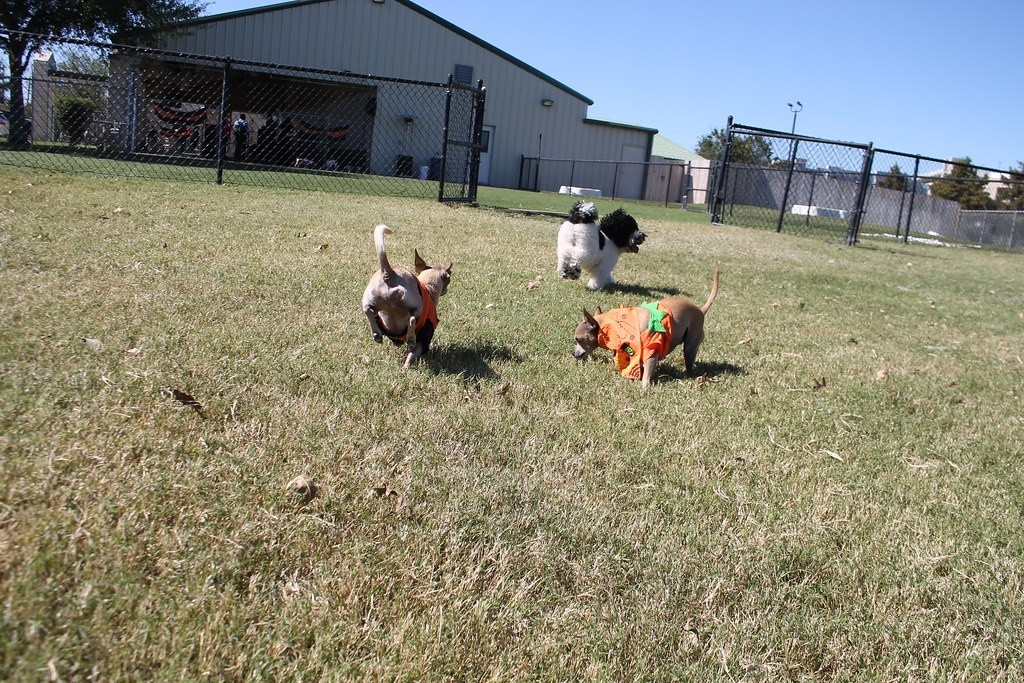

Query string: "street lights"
[[787, 101, 802, 168]]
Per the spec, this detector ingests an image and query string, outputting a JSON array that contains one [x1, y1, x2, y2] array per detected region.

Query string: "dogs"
[[571, 268, 719, 384], [362, 224, 455, 367], [555, 201, 650, 290]]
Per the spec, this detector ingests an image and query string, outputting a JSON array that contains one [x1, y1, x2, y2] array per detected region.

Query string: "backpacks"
[[232, 121, 246, 138]]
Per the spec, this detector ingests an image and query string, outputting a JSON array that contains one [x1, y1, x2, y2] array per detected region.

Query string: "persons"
[[233, 114, 249, 162]]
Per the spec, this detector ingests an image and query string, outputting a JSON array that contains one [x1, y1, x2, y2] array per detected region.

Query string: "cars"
[[4, 111, 31, 135], [0, 111, 10, 137]]
